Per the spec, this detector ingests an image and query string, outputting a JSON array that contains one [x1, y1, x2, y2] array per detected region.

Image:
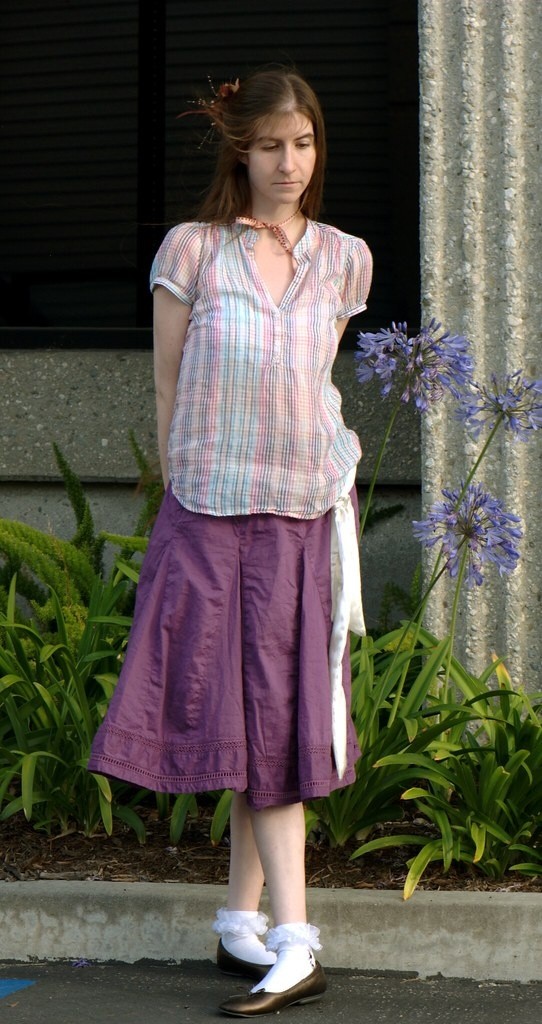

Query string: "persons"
[[87, 69, 373, 1018]]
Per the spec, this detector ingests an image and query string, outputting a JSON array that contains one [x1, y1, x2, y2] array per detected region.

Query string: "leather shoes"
[[218, 960, 329, 1018], [217, 937, 275, 974]]
